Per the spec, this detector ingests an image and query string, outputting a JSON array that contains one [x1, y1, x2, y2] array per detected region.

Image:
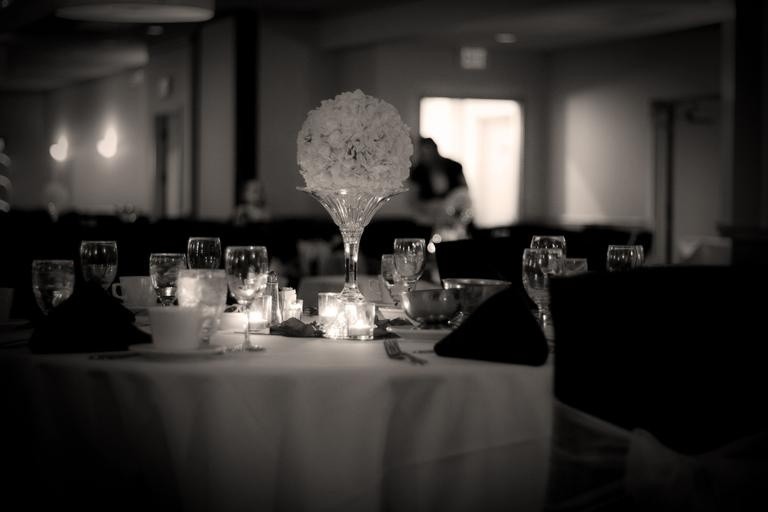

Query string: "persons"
[[405, 138, 474, 227], [234, 181, 273, 225]]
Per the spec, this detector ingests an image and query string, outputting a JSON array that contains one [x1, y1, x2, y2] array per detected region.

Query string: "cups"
[[31, 259, 75, 316], [111, 276, 158, 305], [317, 292, 342, 328], [606, 244, 645, 272], [145, 237, 228, 349], [0, 288, 14, 322], [220, 312, 249, 333], [75, 240, 118, 291], [242, 296, 272, 332], [343, 301, 377, 341]]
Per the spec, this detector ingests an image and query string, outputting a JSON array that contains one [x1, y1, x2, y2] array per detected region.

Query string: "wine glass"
[[224, 246, 269, 354], [393, 237, 427, 292], [521, 235, 588, 354], [381, 254, 401, 303]]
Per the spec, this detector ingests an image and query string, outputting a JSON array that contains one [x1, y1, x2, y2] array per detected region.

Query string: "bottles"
[[265, 270, 283, 327]]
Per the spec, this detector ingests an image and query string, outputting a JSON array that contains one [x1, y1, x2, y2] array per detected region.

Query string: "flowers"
[[296, 89, 415, 209]]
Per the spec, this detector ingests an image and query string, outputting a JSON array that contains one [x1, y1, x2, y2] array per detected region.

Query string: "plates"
[[129, 341, 228, 359], [0, 319, 30, 330], [121, 305, 151, 318], [378, 307, 405, 322]]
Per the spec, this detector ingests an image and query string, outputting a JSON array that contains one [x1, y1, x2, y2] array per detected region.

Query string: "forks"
[[383, 339, 429, 366]]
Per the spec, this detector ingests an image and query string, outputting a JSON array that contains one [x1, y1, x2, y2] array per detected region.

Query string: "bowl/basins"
[[399, 288, 462, 329], [442, 278, 512, 317]]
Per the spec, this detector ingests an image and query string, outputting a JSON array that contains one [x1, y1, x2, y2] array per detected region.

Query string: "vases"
[[294, 185, 410, 305]]
